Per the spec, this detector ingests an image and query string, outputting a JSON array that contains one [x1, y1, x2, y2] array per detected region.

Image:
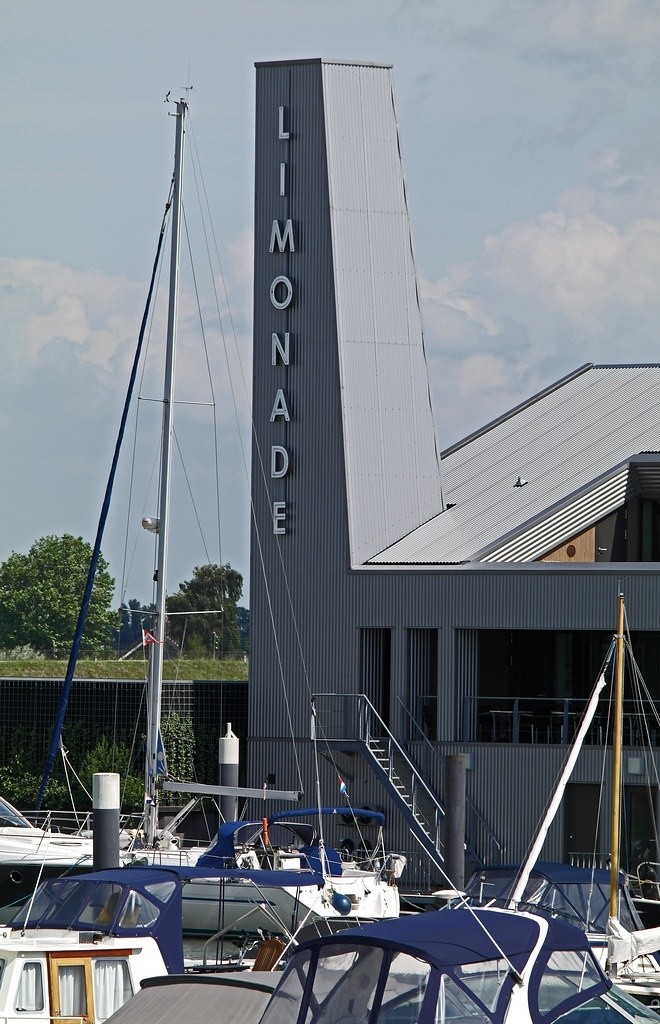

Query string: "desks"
[[490, 709, 535, 744], [549, 711, 602, 745], [623, 713, 660, 747]]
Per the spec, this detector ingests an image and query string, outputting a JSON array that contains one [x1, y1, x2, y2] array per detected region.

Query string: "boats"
[[0, 87, 658, 1022]]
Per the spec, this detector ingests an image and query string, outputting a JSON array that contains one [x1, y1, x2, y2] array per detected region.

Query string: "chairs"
[[97, 893, 143, 931], [251, 938, 287, 971]]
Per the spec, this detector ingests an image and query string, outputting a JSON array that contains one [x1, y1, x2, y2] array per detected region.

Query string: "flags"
[[144, 630, 159, 646]]
